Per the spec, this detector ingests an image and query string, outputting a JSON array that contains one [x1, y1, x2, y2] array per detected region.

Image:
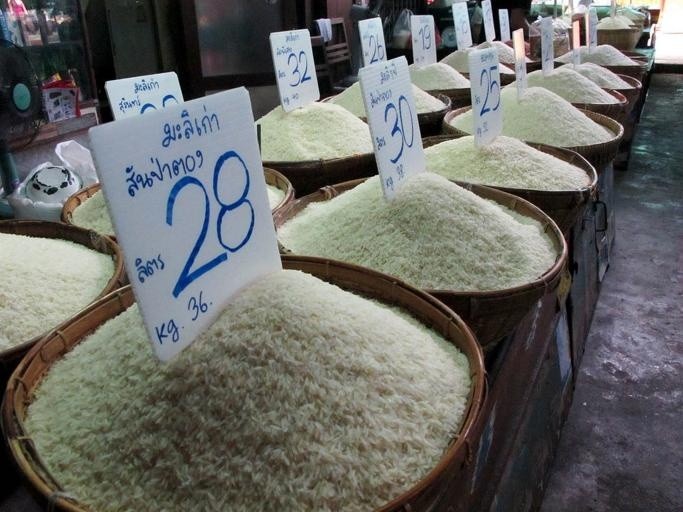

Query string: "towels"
[[316, 18, 332, 42]]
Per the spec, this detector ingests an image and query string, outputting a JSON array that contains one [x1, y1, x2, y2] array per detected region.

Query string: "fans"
[[0, 38, 43, 219]]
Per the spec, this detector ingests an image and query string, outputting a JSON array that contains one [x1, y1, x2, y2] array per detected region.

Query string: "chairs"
[[312, 17, 358, 90], [310, 37, 337, 100]]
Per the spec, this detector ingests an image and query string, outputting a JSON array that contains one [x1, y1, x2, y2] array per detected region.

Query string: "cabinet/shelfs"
[[611, 62, 658, 173], [438, 156, 616, 512]]
